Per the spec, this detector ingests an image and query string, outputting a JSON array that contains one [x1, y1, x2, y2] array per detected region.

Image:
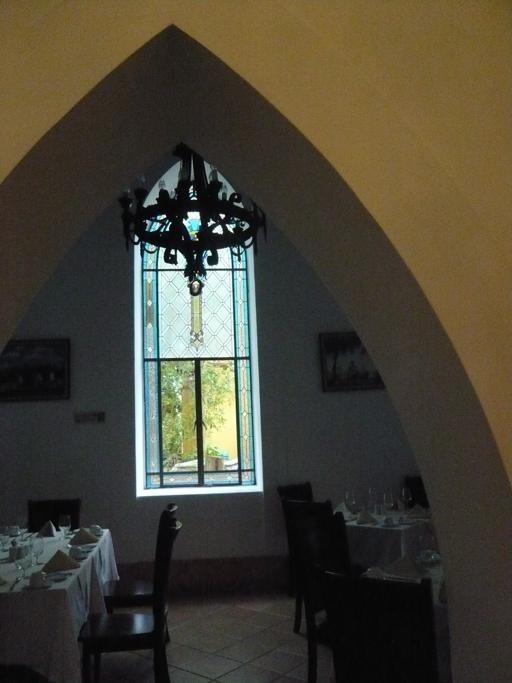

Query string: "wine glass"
[[58, 514, 71, 541], [13, 536, 44, 585], [344, 486, 409, 524]]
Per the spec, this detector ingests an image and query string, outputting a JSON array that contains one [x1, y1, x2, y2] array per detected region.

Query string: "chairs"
[[23, 493, 184, 682], [277, 474, 443, 683]]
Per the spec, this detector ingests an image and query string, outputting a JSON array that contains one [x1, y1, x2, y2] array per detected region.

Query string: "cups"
[[30, 571, 50, 587], [8, 525, 20, 537], [89, 524, 101, 534], [69, 545, 82, 559]]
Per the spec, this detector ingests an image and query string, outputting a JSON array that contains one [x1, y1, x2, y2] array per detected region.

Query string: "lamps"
[[117, 146, 269, 297]]
